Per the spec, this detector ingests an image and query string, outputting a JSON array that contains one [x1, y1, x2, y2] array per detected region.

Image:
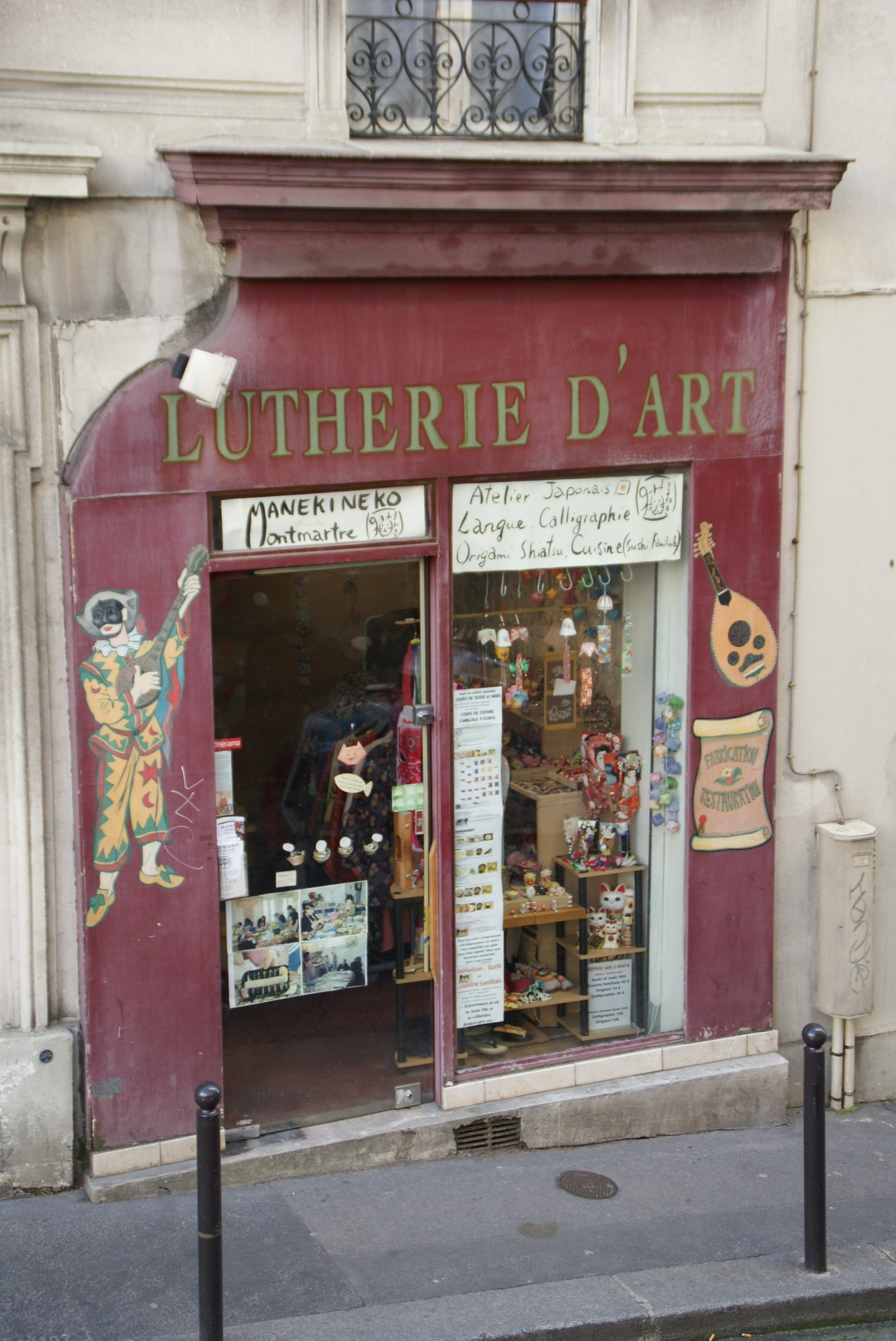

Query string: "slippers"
[[464, 1023, 509, 1055], [492, 1024, 534, 1043]]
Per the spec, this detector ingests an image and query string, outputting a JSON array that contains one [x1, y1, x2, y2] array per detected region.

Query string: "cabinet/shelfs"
[[387, 710, 647, 1077]]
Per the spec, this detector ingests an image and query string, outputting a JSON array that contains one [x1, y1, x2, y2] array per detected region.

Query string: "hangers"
[[304, 687, 398, 758], [410, 606, 554, 652]]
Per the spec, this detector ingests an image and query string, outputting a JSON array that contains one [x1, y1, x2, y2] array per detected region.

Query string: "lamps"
[[171, 348, 236, 408]]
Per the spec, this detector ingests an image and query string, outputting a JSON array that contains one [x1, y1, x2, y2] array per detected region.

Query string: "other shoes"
[[394, 1016, 464, 1058]]
